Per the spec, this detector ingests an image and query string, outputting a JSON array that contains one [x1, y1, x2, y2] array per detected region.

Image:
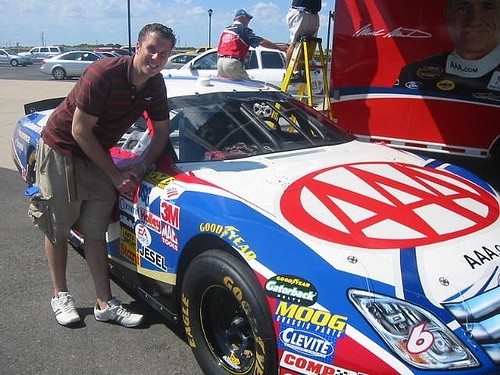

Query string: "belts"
[[220, 53, 242, 61]]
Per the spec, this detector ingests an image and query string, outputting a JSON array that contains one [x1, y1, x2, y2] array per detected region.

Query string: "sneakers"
[[94, 296, 144, 328], [51, 289, 81, 326]]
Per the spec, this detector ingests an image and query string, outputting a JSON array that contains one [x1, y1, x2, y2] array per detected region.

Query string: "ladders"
[[268, 35, 333, 132]]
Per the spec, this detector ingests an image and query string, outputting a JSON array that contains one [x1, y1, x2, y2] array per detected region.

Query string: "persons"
[[282, 0, 321, 82], [393, 0, 500, 101], [216, 9, 289, 79], [28, 23, 176, 328]]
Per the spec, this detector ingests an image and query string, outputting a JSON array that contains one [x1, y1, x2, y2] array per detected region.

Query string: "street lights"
[[207, 8, 213, 49]]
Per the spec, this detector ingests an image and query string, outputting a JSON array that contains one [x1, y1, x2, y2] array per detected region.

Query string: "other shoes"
[[283, 72, 304, 82]]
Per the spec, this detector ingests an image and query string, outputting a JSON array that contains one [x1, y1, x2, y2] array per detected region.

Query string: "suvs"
[[17, 46, 66, 62]]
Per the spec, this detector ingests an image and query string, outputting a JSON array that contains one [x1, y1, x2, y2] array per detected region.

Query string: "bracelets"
[[138, 159, 149, 173]]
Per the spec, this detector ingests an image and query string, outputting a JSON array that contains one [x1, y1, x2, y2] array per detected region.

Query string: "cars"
[[12, 75, 499, 374], [0, 42, 323, 95]]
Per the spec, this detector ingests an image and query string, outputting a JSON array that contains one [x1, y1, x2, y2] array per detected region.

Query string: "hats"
[[236, 9, 253, 20]]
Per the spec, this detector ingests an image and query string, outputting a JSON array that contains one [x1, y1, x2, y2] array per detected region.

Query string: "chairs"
[[179, 111, 260, 161]]
